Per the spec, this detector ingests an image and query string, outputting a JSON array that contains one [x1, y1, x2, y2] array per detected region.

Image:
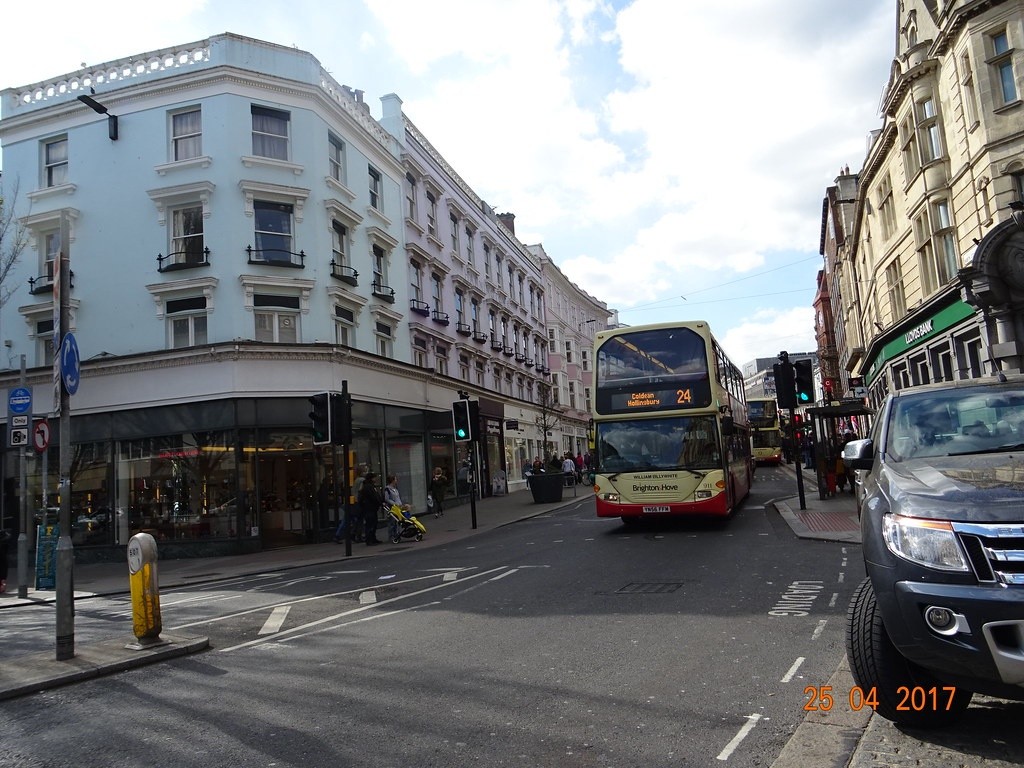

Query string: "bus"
[[745, 399, 781, 467], [593, 319, 754, 529]]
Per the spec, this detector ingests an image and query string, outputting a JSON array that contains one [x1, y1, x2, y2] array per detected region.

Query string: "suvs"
[[842, 373, 1023, 732]]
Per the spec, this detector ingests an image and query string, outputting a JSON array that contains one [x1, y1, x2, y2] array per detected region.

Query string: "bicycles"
[[580, 466, 595, 486]]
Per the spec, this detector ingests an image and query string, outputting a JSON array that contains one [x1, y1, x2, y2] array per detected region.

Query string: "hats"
[[365, 472, 376, 479]]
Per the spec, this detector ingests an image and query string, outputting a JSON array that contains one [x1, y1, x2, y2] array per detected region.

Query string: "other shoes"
[[351, 535, 360, 543], [332, 537, 342, 544], [366, 538, 383, 546], [526, 487, 528, 491]]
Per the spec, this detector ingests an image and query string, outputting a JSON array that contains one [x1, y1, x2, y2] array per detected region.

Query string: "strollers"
[[382, 505, 426, 544]]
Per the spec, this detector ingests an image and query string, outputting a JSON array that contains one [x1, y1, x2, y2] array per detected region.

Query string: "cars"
[[80, 506, 113, 525], [212, 497, 239, 515], [34, 507, 60, 525]]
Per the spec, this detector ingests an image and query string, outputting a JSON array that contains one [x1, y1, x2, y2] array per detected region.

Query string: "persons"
[[782, 429, 858, 493], [401, 504, 412, 520], [524, 449, 594, 491], [332, 473, 388, 546], [385, 475, 404, 541], [428, 467, 447, 518]]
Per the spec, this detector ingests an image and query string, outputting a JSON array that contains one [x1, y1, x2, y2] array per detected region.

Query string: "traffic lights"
[[453, 400, 471, 443], [308, 391, 331, 445], [794, 414, 803, 424], [468, 400, 484, 442], [332, 392, 355, 446], [793, 358, 817, 406], [795, 430, 802, 440]]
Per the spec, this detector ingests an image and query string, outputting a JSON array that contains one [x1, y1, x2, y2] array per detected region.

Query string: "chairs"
[[962, 423, 991, 441], [907, 403, 955, 454]]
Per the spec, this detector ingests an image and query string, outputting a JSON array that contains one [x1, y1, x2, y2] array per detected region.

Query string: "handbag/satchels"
[[836, 457, 845, 474], [427, 495, 434, 507]]
